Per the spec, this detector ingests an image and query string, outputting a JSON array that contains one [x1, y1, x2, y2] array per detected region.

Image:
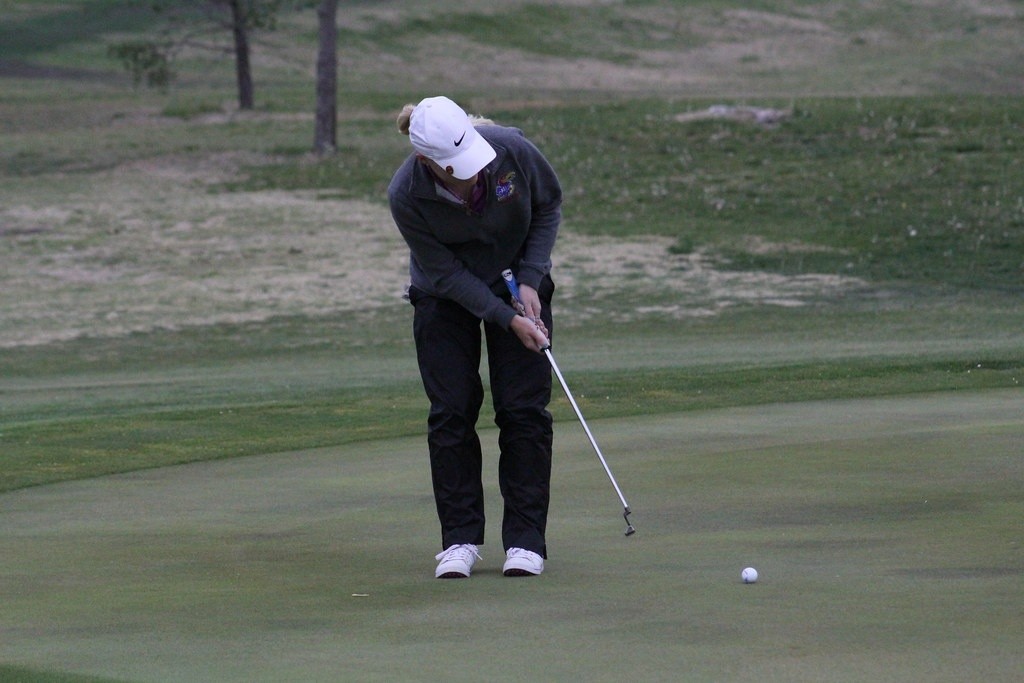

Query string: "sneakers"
[[435, 543, 482, 578], [503, 547, 544, 576]]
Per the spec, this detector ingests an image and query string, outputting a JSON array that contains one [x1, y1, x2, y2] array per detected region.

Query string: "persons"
[[388, 96, 563, 578]]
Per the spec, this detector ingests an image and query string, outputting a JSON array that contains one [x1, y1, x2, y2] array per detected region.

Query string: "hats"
[[408, 96, 497, 180]]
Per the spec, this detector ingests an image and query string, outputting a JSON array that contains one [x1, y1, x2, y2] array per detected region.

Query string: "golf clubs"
[[502, 267, 637, 537]]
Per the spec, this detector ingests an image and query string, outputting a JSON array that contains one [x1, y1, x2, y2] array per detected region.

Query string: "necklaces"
[[445, 184, 471, 206]]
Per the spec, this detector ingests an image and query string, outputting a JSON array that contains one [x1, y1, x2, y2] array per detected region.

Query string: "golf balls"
[[741, 567, 758, 584]]
